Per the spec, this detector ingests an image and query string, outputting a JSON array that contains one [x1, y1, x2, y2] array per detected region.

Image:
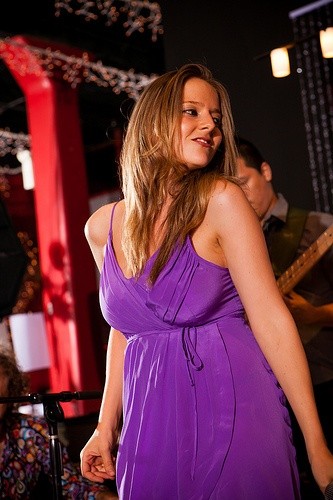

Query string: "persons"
[[221, 138, 332, 500], [0, 353, 119, 500], [79, 63, 332, 500]]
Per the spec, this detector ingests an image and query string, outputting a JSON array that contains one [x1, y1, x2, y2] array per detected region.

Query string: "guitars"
[[245, 224, 333, 325]]
